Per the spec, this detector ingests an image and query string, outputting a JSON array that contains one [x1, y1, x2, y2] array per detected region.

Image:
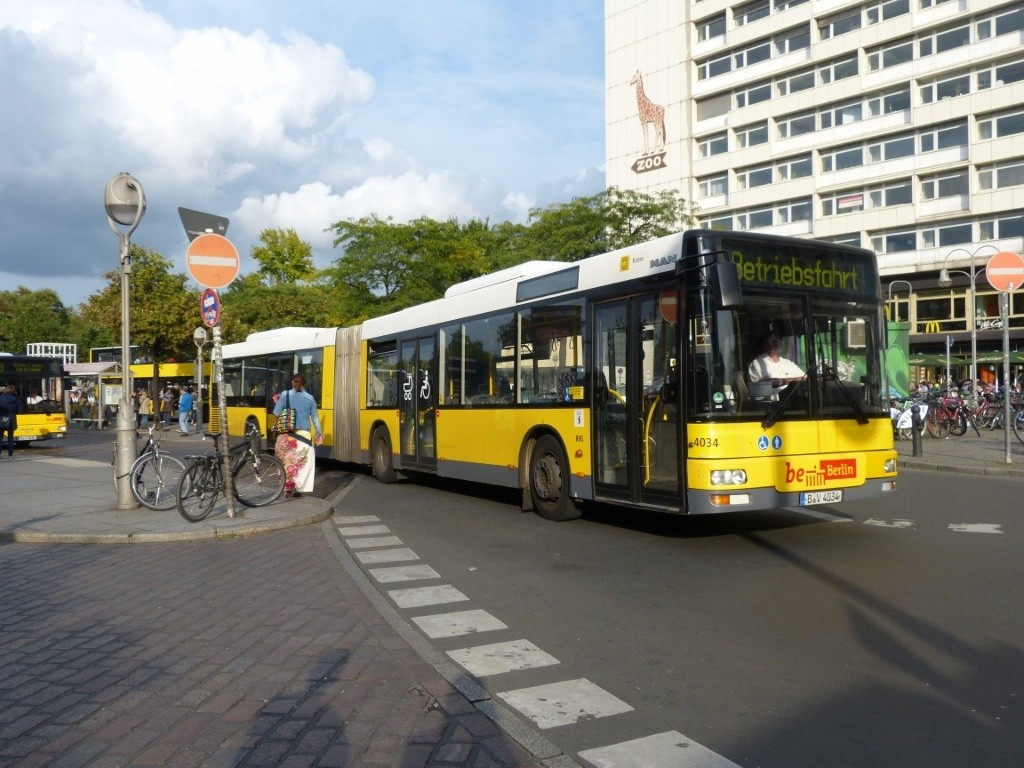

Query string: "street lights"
[[938, 245, 1001, 409]]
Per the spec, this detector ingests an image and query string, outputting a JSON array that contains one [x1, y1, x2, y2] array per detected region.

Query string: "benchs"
[[73, 418, 107, 422]]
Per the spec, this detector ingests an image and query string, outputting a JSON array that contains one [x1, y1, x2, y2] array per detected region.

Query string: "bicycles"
[[176, 421, 287, 523], [883, 374, 1024, 446], [110, 414, 191, 511]]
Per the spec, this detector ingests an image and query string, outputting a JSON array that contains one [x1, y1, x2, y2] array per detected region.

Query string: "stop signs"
[[186, 233, 239, 289], [985, 251, 1024, 292]]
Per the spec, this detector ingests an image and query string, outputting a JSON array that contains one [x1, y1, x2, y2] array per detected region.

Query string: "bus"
[[89, 342, 210, 424], [0, 351, 73, 449], [207, 227, 899, 523]]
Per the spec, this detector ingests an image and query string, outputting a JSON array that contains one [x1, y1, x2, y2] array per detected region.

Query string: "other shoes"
[[284, 491, 291, 497], [8, 456, 13, 460], [293, 492, 301, 498], [180, 433, 189, 436]]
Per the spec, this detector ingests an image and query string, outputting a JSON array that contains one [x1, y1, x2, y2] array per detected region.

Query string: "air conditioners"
[[843, 317, 866, 349]]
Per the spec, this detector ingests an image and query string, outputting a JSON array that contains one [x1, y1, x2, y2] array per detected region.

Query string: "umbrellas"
[[908, 352, 1024, 392]]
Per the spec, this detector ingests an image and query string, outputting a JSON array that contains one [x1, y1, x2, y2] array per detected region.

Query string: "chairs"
[[252, 382, 267, 396], [446, 372, 583, 404]]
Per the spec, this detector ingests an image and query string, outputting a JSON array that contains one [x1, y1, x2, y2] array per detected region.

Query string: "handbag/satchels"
[[0, 416, 10, 429], [271, 390, 296, 433]]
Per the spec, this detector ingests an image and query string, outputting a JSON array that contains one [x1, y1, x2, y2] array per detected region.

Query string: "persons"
[[71, 382, 197, 435], [749, 331, 808, 402], [273, 375, 323, 497], [0, 385, 21, 460], [134, 354, 176, 364], [909, 381, 997, 398], [27, 390, 42, 405]]
